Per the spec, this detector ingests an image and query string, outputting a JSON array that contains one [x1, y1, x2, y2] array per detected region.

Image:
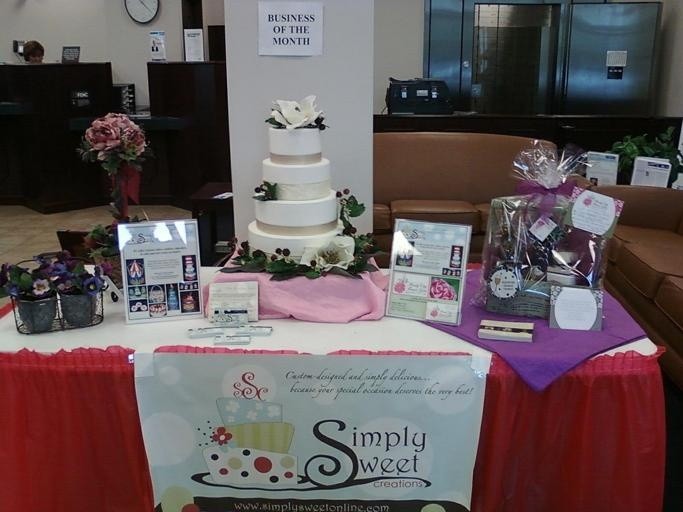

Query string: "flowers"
[[2, 249, 114, 302], [76, 113, 151, 175]]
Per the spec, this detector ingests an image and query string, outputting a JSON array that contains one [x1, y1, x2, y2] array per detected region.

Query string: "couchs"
[[591, 185, 682, 424], [373, 133, 592, 268]]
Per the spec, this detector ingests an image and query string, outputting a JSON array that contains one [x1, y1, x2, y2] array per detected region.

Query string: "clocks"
[[123, 0, 160, 24]]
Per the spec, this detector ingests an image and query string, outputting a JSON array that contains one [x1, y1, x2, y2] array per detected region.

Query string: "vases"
[[112, 170, 130, 223], [12, 289, 98, 333]]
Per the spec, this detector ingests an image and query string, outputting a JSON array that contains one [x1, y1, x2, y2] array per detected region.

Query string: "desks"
[[0, 260, 660, 512]]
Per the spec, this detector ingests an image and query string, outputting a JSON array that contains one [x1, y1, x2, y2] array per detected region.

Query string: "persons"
[[151, 40, 158, 52], [22, 41, 43, 65]]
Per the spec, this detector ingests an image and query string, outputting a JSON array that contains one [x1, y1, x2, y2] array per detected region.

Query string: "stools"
[[188, 182, 233, 266]]
